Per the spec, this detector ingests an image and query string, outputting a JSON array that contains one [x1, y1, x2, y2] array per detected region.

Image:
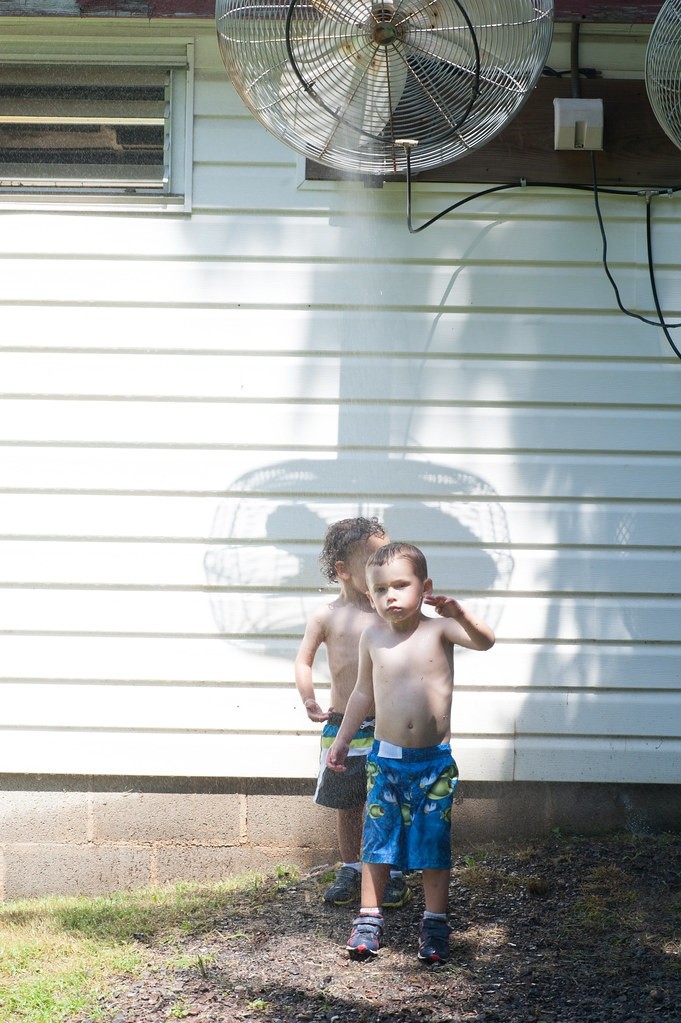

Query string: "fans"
[[644, 0, 681, 150], [216, 0, 554, 176]]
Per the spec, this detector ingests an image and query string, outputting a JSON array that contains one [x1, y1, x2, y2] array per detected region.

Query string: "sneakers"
[[381, 874, 412, 907], [345, 912, 385, 957], [322, 866, 362, 905], [418, 917, 453, 961]]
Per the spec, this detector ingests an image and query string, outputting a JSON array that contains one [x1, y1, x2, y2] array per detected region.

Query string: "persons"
[[294, 517, 412, 907], [326, 543, 495, 961]]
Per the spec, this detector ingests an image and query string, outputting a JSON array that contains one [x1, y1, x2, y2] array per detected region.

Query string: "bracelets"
[[304, 699, 316, 704]]
